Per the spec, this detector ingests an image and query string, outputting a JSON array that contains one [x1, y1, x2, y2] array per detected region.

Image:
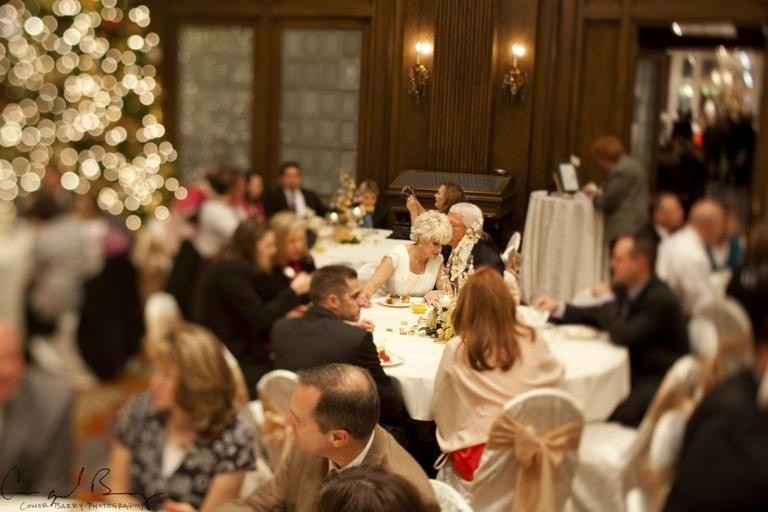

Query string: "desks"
[[384, 170, 517, 232], [520, 188, 612, 313]]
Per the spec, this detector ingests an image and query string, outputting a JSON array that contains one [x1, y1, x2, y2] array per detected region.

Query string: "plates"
[[379, 346, 404, 367], [378, 296, 426, 307]]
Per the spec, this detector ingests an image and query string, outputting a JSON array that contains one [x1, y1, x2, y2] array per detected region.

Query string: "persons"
[[0, 90, 767, 512]]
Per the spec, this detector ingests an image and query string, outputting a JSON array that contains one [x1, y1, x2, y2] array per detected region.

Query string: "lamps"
[[499, 43, 529, 103], [405, 41, 430, 104]]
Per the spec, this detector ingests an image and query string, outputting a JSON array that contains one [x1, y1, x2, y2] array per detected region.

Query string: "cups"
[[411, 301, 427, 314]]
